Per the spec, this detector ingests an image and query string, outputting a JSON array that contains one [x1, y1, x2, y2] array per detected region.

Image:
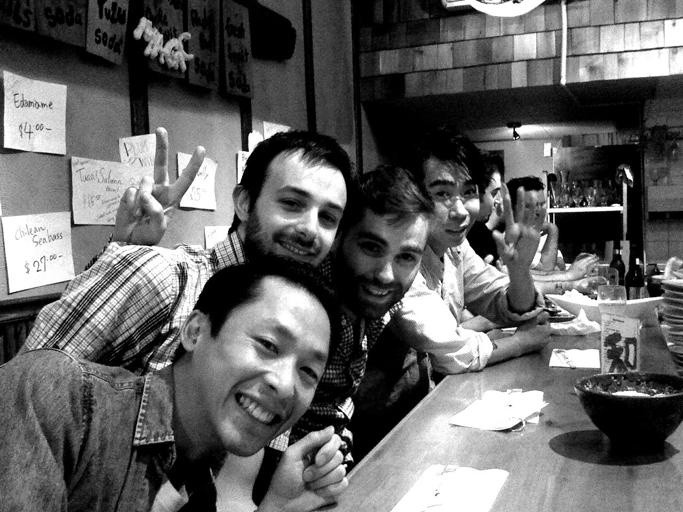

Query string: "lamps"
[[507, 121, 521, 140]]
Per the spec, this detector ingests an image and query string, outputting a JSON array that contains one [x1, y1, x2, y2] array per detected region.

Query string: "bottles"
[[625, 263, 644, 300], [609, 246, 626, 286]]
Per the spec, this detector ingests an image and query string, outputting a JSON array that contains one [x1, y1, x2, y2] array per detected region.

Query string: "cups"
[[586, 263, 610, 294], [597, 285, 627, 317], [550, 181, 609, 208]]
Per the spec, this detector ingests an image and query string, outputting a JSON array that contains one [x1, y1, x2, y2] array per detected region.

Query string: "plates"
[[544, 292, 664, 327], [661, 277, 683, 369]]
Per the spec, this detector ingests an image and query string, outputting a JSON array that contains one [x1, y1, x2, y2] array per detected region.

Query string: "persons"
[[12, 128, 353, 461], [353, 123, 553, 472], [62, 128, 436, 471], [0, 253, 349, 512], [466, 153, 566, 275]]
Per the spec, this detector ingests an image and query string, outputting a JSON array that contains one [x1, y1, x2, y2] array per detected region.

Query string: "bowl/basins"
[[574, 370, 683, 448]]
